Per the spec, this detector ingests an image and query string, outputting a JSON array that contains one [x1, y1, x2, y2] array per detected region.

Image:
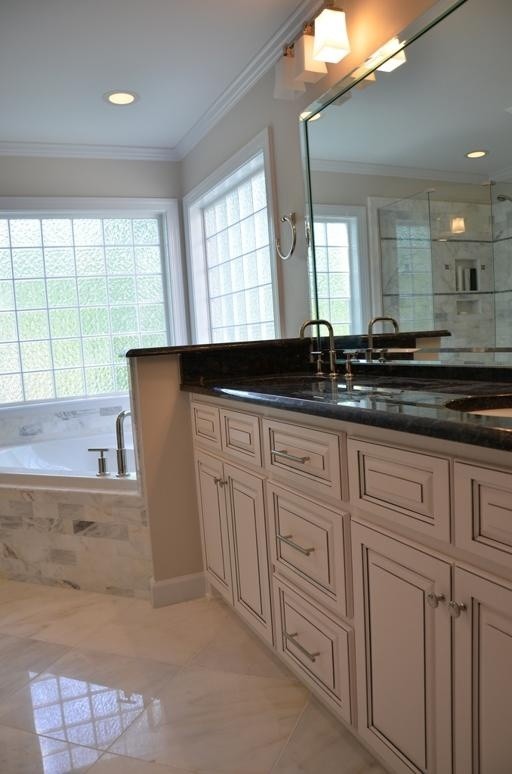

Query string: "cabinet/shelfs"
[[180, 400, 271, 649], [263, 417, 351, 738], [348, 438, 509, 774]]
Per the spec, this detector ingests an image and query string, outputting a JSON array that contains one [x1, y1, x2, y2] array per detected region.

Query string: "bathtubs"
[[0, 431, 141, 489]]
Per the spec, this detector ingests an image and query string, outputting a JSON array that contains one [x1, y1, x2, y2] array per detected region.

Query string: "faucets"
[[366, 316, 399, 359], [116, 410, 132, 476], [300, 320, 338, 377]]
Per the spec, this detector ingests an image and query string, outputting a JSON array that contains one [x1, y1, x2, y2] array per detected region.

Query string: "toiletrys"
[[457, 265, 477, 292]]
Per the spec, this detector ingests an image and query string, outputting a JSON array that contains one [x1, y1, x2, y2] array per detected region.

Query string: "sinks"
[[446, 394, 512, 418]]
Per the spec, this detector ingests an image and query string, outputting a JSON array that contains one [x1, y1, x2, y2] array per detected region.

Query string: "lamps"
[[272, 0, 354, 102]]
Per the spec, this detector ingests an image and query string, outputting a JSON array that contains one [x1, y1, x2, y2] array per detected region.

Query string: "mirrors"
[[295, 0, 512, 347]]
